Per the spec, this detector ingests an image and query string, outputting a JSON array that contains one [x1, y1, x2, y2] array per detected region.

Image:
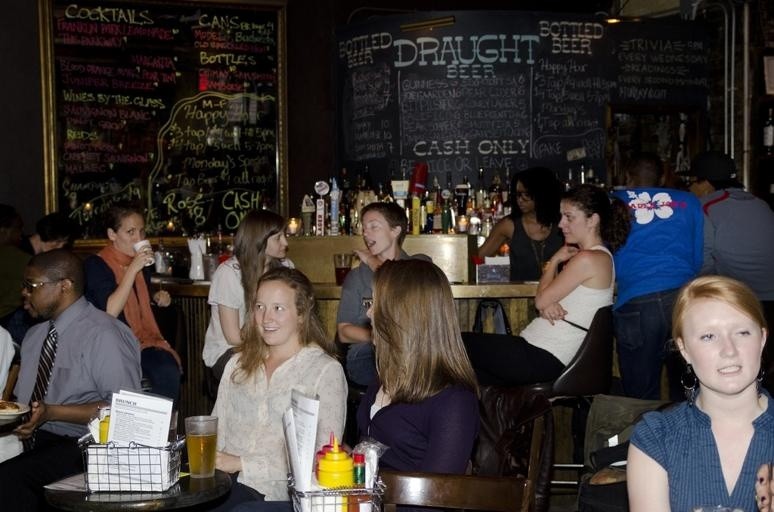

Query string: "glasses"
[[362, 297, 374, 308], [515, 191, 532, 201], [19, 278, 75, 294], [684, 180, 697, 187]]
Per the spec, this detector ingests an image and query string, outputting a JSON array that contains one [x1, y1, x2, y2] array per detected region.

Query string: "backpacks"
[[471, 385, 553, 478]]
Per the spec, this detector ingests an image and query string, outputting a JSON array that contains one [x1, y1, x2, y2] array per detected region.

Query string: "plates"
[[0, 402, 30, 419]]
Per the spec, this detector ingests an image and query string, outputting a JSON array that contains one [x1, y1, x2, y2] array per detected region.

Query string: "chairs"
[[581, 392, 672, 506], [554, 304, 636, 391], [378, 388, 557, 506]]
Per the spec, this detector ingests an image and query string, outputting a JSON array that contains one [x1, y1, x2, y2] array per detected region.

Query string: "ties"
[[21, 325, 58, 451]]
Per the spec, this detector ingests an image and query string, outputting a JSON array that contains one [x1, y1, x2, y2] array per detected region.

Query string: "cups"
[[134, 239, 156, 267], [185, 416, 219, 479], [202, 255, 220, 281], [334, 254, 353, 286]]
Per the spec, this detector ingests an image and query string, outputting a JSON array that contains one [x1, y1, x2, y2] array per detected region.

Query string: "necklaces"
[[525, 215, 544, 270]]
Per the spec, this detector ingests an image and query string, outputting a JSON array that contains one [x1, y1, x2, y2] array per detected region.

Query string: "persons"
[[209, 267, 349, 511], [2, 253, 141, 504], [609, 153, 704, 396], [478, 165, 565, 283], [86, 200, 182, 401], [684, 154, 772, 393], [626, 274, 773, 512], [334, 202, 431, 387], [458, 183, 631, 387], [2, 203, 69, 402], [202, 209, 296, 378], [358, 258, 482, 512]]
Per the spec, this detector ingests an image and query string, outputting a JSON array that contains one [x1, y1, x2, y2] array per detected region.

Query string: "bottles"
[[316, 431, 372, 512], [554, 164, 600, 193], [341, 168, 350, 191], [421, 166, 512, 258], [154, 240, 168, 273], [205, 231, 236, 255]]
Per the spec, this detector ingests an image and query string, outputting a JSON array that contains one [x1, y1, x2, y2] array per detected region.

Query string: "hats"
[[674, 151, 737, 181]]
